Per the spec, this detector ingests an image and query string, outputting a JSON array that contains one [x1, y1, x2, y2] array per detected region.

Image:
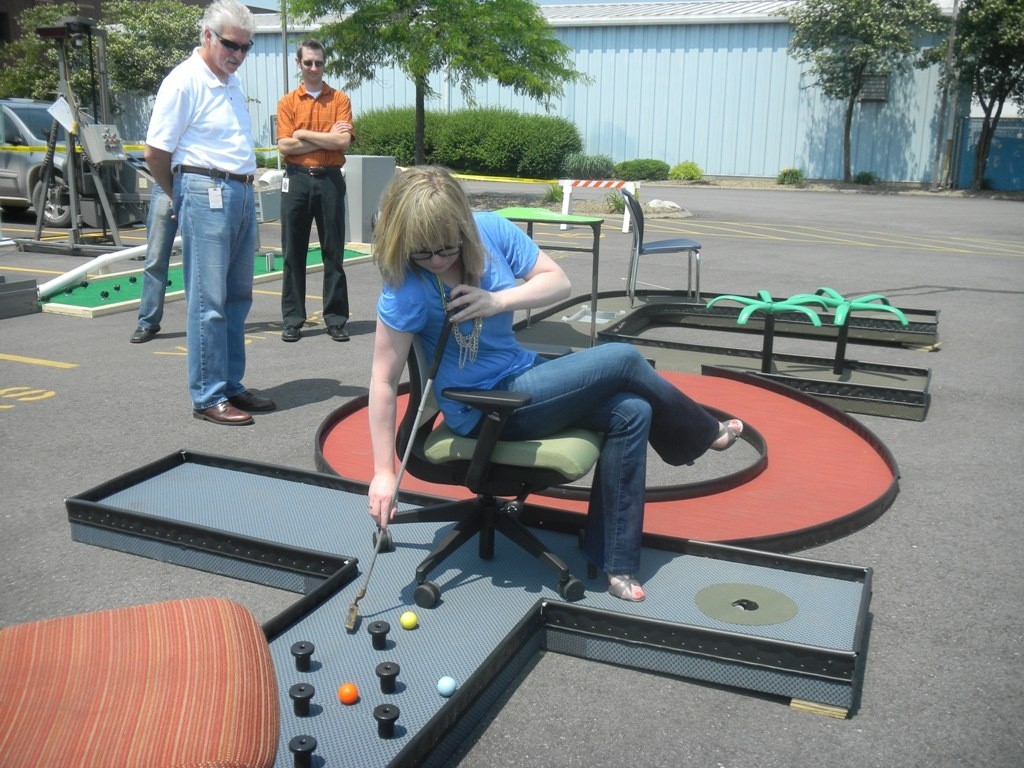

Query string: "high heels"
[[686, 419, 743, 466], [587, 562, 645, 601]]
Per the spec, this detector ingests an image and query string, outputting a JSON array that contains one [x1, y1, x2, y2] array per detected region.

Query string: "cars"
[[0, 98, 126, 228]]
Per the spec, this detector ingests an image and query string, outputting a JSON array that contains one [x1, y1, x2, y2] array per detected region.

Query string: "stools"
[[0, 598, 280, 768]]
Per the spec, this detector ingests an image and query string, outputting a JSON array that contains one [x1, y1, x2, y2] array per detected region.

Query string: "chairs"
[[621, 186, 703, 308], [371, 211, 605, 609]]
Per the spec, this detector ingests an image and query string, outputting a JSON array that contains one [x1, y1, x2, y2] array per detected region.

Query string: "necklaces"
[[435, 260, 483, 370]]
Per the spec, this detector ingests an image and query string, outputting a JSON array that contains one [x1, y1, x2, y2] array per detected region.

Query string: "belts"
[[286, 164, 340, 175], [173, 165, 254, 184]]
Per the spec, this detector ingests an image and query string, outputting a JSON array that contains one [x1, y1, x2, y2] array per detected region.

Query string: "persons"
[[369, 168, 743, 601], [130, 181, 181, 344], [276, 37, 355, 341], [145, 1, 277, 426]]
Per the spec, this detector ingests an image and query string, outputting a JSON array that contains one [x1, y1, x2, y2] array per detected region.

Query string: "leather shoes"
[[327, 325, 350, 341], [228, 391, 276, 411], [193, 401, 254, 425], [130, 326, 160, 342], [282, 325, 301, 340]]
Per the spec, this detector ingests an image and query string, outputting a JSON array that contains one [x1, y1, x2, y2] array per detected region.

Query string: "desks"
[[493, 205, 604, 348]]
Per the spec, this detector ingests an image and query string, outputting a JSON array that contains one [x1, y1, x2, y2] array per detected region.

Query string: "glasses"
[[409, 231, 463, 260], [301, 60, 324, 67], [209, 28, 254, 53]]
